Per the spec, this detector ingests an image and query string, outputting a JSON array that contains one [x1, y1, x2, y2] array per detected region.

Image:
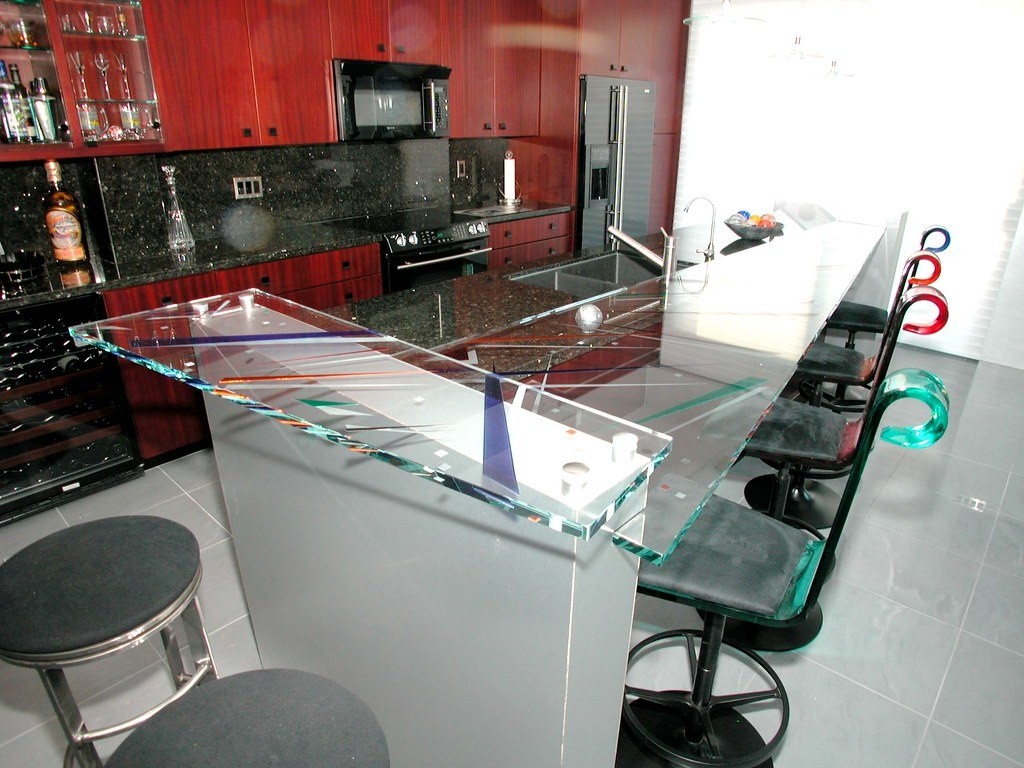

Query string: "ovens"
[[382, 237, 488, 295]]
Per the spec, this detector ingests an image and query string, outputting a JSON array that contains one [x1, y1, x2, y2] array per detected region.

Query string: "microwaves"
[[334, 60, 453, 141]]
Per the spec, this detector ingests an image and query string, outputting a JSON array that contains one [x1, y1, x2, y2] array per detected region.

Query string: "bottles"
[[161, 165, 198, 268], [0, 291, 135, 506], [0, 59, 63, 143], [44, 161, 91, 290]]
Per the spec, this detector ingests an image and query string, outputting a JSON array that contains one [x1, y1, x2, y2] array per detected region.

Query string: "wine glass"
[[79, 10, 94, 34], [71, 53, 132, 100]]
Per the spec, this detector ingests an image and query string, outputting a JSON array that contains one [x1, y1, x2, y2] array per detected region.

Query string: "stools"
[[102, 668, 391, 768], [0, 516, 220, 768]]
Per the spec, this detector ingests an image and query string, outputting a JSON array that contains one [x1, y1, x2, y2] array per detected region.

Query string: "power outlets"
[[233, 176, 263, 200], [457, 159, 466, 178]]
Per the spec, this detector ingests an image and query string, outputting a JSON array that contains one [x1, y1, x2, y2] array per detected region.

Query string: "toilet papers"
[[503, 158, 516, 201]]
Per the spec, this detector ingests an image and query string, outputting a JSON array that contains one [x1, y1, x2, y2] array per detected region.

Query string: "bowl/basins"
[[724, 219, 784, 241]]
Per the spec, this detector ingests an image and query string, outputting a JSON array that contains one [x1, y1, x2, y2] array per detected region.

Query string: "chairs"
[[816, 224, 951, 412], [696, 284, 949, 653], [743, 250, 942, 530], [613, 367, 950, 768]]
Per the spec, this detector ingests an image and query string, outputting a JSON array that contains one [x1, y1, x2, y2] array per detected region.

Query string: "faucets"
[[684, 195, 717, 262], [605, 223, 680, 276]]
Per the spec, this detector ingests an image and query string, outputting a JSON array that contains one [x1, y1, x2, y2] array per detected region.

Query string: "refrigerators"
[[576, 75, 656, 257]]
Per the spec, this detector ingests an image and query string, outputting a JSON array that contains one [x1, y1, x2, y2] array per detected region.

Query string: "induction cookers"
[[320, 206, 490, 255]]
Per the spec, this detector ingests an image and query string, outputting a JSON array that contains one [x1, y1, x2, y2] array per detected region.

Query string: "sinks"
[[565, 252, 699, 287], [509, 269, 628, 299]]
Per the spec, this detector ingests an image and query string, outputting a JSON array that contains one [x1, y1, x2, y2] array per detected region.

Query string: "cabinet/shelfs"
[[0, 0, 164, 161], [328, 0, 441, 66], [101, 245, 384, 463], [489, 213, 574, 272], [141, 0, 338, 152], [440, 0, 541, 138], [509, 0, 684, 251]]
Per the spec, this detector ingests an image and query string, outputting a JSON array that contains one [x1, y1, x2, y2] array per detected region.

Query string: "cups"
[[8, 17, 40, 50], [117, 103, 155, 140], [97, 6, 129, 37], [76, 105, 109, 141], [60, 8, 77, 33]]
